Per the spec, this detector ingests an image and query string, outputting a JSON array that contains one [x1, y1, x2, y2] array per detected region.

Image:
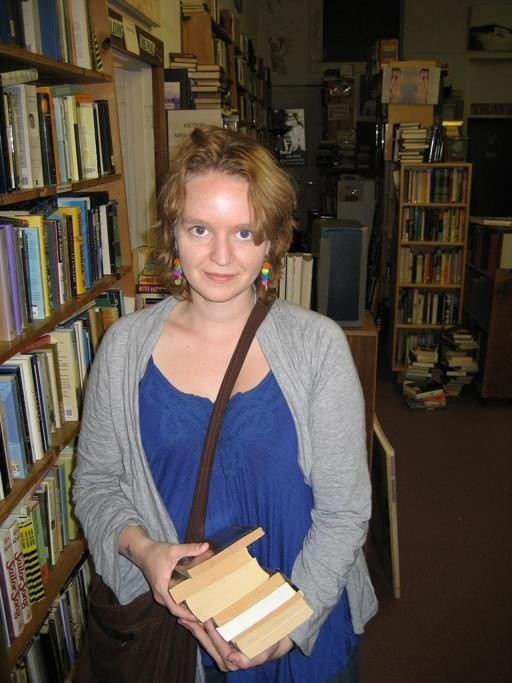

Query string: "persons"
[[72, 127, 379, 682], [388, 64, 436, 104]]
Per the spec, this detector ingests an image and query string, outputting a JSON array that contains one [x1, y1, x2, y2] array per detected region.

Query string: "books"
[[393, 122, 429, 163], [402, 207, 465, 243], [0, 191, 123, 341], [0, 0, 100, 70], [169, 52, 227, 110], [0, 288, 124, 500], [0, 432, 79, 648], [181, 0, 227, 72], [11, 558, 91, 683], [278, 253, 314, 311], [168, 524, 315, 660], [0, 68, 121, 194], [400, 247, 462, 285], [398, 328, 480, 409], [398, 288, 458, 326], [135, 265, 171, 308], [404, 167, 468, 202]]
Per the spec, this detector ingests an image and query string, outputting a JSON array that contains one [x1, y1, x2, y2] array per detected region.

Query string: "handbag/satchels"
[[72, 288, 277, 682]]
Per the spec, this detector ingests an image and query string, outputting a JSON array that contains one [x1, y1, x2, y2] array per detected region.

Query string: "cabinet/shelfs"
[[465, 221, 512, 402], [373, 57, 475, 373], [167, 0, 274, 170], [1, 1, 168, 683]]
